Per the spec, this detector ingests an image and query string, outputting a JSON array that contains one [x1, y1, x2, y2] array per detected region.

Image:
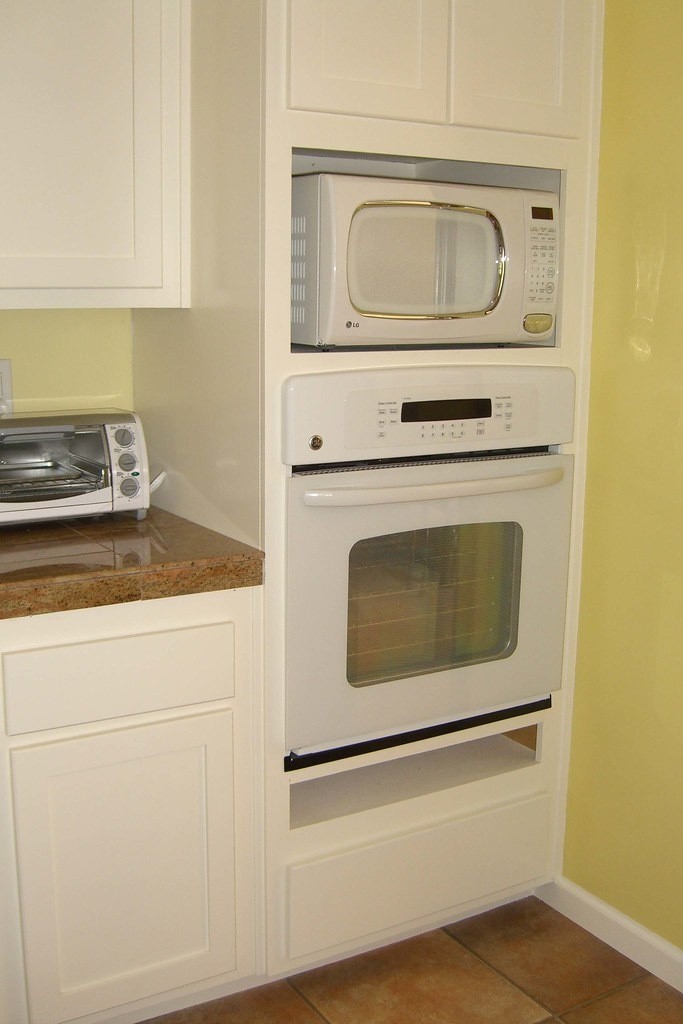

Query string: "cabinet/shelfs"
[[279, 0, 583, 140], [262, 690, 566, 985], [0, 585, 263, 1024], [0, 0, 193, 310]]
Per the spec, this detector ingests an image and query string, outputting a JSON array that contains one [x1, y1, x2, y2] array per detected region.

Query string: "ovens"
[[285, 368, 575, 773], [0, 407, 150, 526]]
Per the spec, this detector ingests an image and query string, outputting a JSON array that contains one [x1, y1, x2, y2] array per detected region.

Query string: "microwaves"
[[293, 172, 559, 345]]
